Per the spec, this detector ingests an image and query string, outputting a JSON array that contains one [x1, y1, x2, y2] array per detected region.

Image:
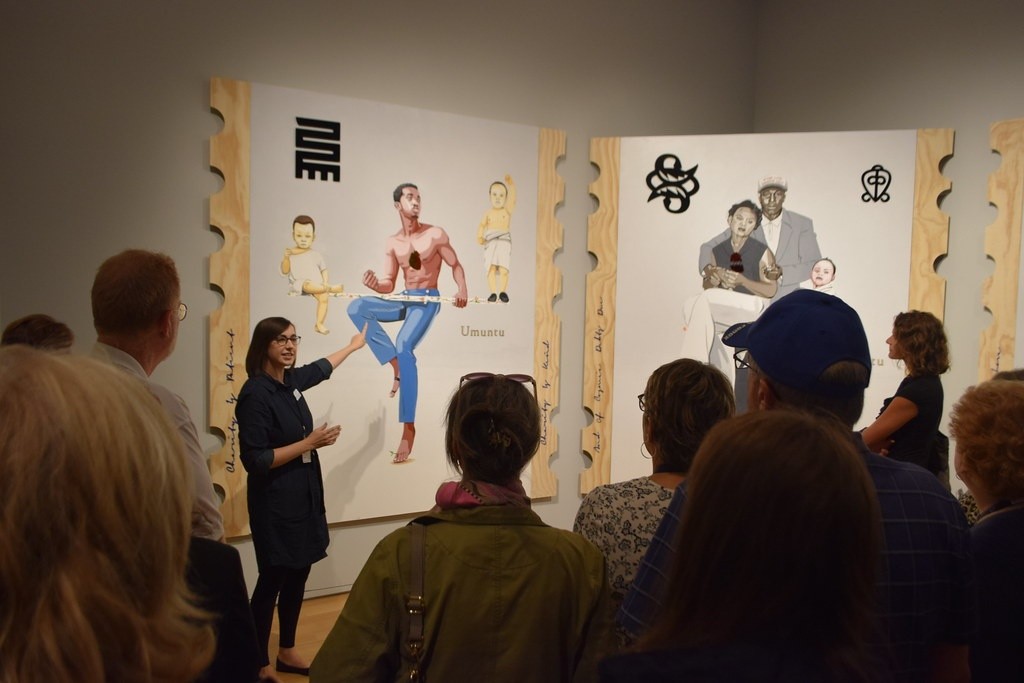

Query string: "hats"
[[722, 289, 871, 397]]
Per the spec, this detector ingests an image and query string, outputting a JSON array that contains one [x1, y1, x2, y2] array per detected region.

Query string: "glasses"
[[734, 349, 753, 369], [277, 336, 301, 346], [638, 394, 647, 411], [460, 373, 537, 404], [158, 303, 187, 321]]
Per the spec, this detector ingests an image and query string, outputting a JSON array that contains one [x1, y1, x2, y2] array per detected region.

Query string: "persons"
[[573, 290, 1024, 683], [309, 373, 617, 683], [0, 249, 261, 683], [235, 316, 368, 683]]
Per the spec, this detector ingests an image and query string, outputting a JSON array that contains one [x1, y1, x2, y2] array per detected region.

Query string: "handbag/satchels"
[[880, 398, 949, 476]]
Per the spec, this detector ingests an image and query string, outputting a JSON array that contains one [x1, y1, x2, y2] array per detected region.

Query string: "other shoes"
[[276, 655, 310, 676]]
